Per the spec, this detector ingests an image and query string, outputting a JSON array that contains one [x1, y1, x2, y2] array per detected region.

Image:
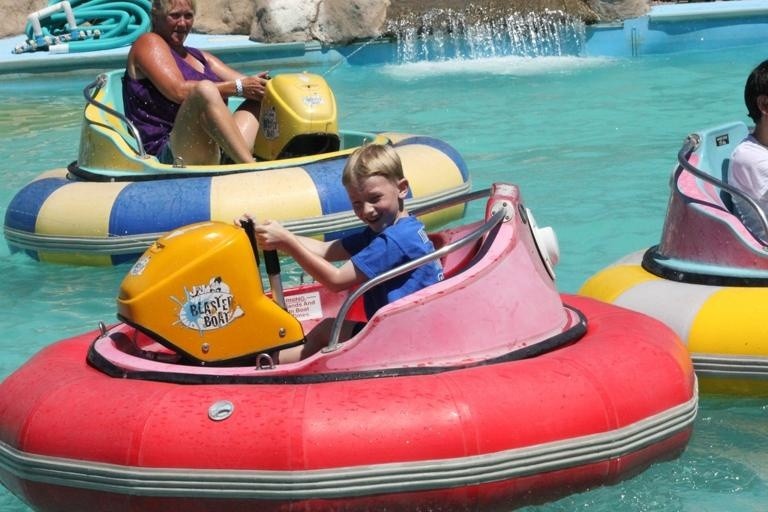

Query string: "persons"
[[229, 142, 445, 368], [118, 1, 274, 168], [725, 61, 768, 253]]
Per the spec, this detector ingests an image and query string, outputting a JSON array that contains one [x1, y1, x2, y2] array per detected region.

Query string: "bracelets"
[[234, 78, 244, 98]]
